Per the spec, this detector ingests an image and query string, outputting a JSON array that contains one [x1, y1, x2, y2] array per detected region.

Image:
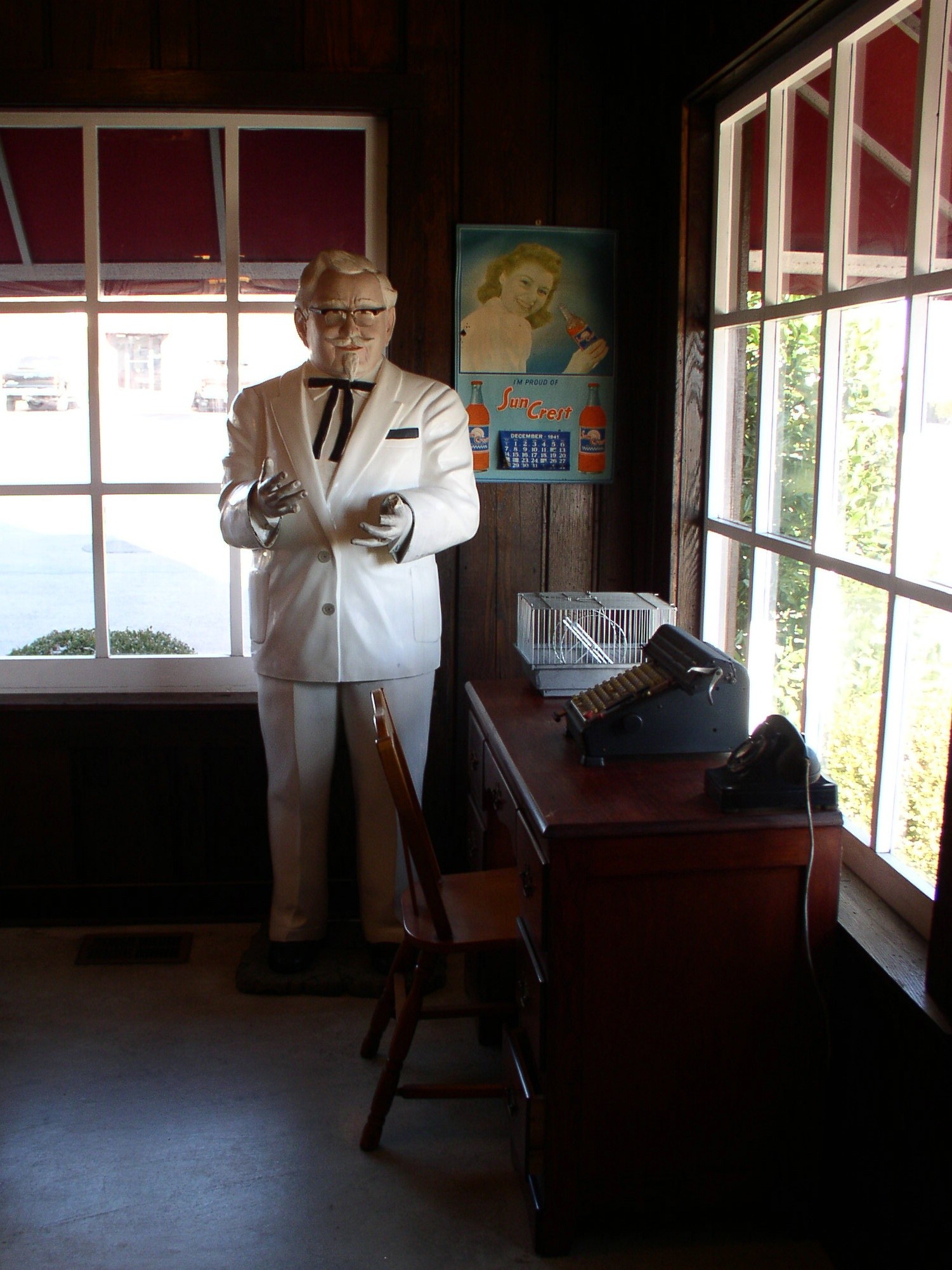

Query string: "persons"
[[218, 249, 482, 985]]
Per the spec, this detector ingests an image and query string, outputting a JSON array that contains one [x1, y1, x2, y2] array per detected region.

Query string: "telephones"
[[705, 714, 838, 810]]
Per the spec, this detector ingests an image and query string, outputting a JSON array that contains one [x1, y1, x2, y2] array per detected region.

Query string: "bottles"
[[465, 380, 491, 472], [578, 383, 606, 474], [558, 301, 599, 351]]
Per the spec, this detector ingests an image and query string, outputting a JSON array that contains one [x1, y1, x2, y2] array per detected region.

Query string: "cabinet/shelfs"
[[465, 676, 848, 1258]]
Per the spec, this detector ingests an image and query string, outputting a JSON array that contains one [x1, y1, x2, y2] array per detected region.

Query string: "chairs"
[[361, 686, 523, 1154]]
